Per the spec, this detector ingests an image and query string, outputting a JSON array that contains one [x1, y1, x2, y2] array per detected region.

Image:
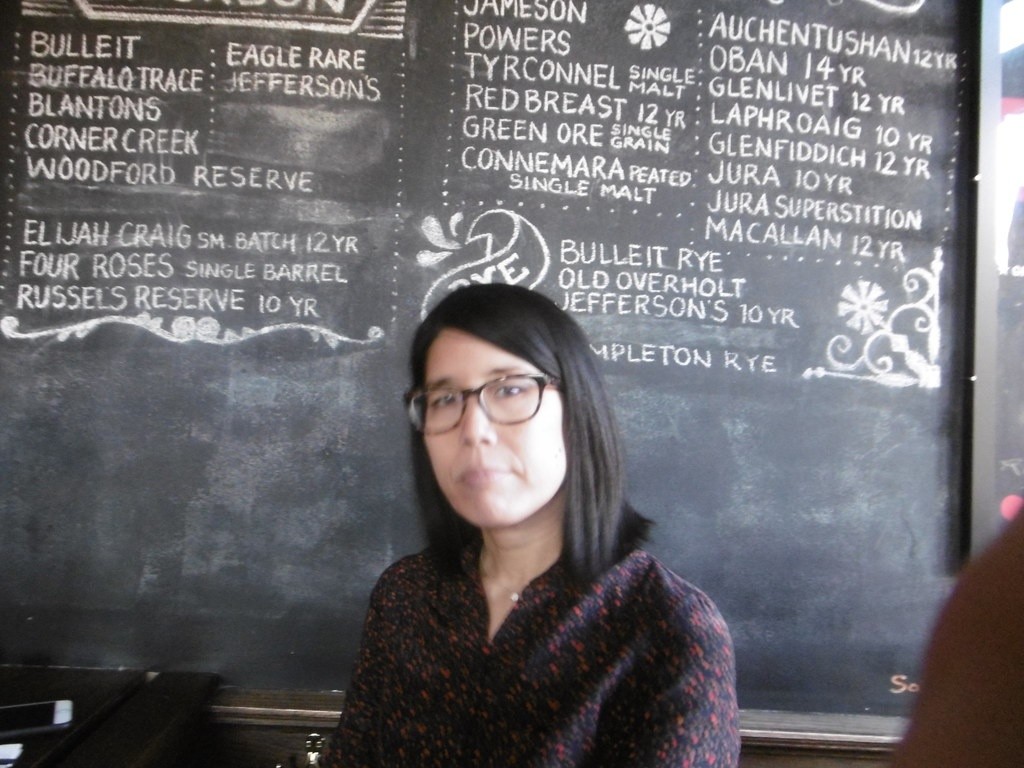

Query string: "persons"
[[318, 281, 743, 767]]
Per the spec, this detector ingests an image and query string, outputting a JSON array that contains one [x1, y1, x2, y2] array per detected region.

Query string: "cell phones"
[[0, 699, 75, 739]]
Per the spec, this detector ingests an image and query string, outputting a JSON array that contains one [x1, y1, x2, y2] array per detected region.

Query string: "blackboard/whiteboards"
[[0, 0, 1003, 752]]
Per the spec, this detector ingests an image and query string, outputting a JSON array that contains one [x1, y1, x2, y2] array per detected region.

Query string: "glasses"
[[402, 371, 561, 434]]
